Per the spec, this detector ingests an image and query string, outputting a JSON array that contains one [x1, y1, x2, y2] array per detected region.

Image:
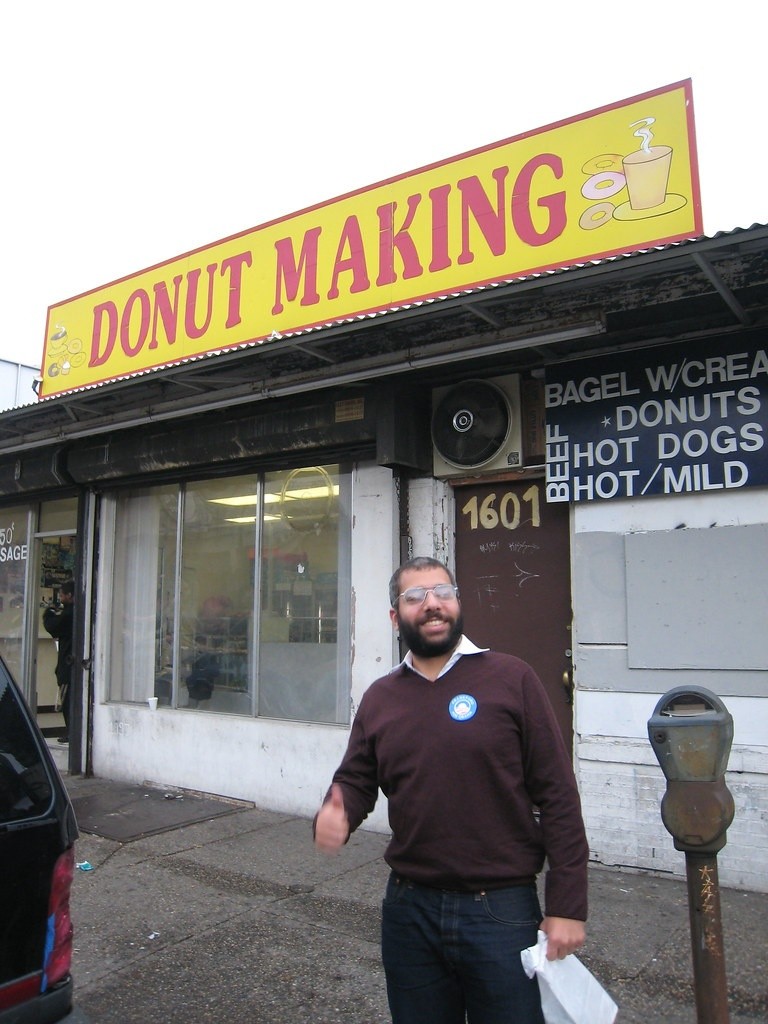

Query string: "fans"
[[432, 373, 524, 480]]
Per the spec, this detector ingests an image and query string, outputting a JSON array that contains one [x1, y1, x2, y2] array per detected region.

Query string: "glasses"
[[392, 584, 460, 606]]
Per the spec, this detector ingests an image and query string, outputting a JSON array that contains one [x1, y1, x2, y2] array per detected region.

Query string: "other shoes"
[[57, 736, 69, 742]]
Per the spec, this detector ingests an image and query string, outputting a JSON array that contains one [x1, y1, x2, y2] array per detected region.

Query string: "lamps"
[[0, 308, 610, 456], [31, 375, 43, 395]]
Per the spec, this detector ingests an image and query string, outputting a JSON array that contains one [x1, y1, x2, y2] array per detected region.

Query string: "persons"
[[155, 589, 173, 705], [42, 582, 74, 743], [310, 556, 590, 1024], [185, 636, 219, 709]]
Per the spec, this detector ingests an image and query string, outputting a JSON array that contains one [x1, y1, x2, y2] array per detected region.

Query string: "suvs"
[[0, 654, 79, 1024]]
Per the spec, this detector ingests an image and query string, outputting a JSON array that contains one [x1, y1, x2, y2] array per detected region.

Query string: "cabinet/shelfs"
[[272, 584, 338, 642], [163, 614, 247, 692]]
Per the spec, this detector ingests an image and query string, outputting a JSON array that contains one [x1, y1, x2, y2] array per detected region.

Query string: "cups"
[[148, 697, 158, 710]]
